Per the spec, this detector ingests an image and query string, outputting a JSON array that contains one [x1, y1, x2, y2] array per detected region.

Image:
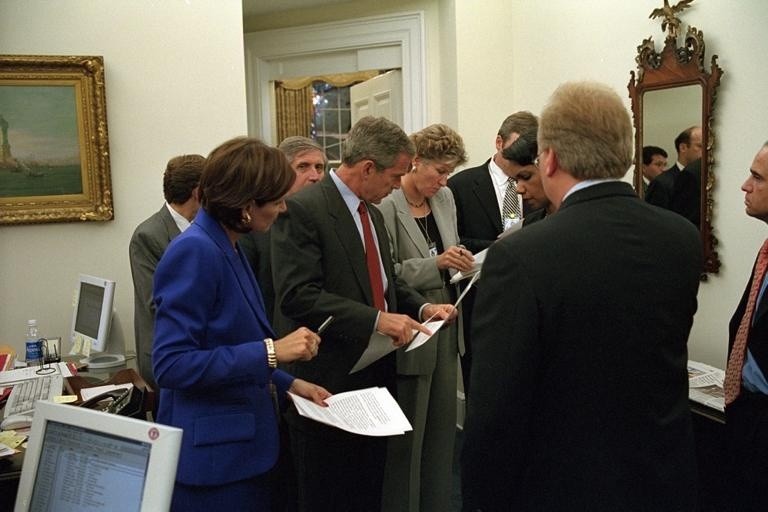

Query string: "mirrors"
[[627, 1, 726, 282]]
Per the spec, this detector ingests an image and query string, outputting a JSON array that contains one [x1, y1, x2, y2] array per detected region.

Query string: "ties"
[[501, 178, 522, 232], [718, 241, 763, 407], [358, 202, 388, 315]]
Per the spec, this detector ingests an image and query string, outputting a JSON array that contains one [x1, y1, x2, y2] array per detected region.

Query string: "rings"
[[312, 344, 315, 353], [404, 195, 427, 209], [313, 339, 317, 344]]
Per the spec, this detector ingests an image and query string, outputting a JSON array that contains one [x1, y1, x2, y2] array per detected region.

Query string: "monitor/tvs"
[[14, 400, 184, 512], [70, 273, 126, 369]]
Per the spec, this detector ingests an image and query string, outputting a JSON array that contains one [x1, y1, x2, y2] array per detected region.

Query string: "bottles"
[[26, 319, 43, 366]]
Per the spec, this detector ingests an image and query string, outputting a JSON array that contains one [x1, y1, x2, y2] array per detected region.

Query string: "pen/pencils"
[[287, 315, 338, 368], [454, 243, 466, 264]]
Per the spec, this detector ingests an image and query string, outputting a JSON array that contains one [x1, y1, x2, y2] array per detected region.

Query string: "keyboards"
[[0, 374, 63, 431]]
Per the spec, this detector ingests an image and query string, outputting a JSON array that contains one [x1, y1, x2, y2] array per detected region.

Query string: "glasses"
[[534, 148, 551, 169]]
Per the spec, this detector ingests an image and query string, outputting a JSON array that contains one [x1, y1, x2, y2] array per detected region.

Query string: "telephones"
[[71, 385, 147, 414]]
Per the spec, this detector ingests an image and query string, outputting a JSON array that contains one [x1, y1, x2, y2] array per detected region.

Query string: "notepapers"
[[70, 336, 84, 355], [83, 340, 99, 360]]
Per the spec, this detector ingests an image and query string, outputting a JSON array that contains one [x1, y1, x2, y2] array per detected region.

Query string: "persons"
[[644, 126, 702, 207], [461, 88, 701, 511], [724, 142, 768, 512], [642, 145, 669, 194], [271, 116, 457, 512], [129, 156, 206, 410], [373, 125, 475, 511], [674, 158, 700, 229], [152, 138, 333, 512], [448, 113, 538, 415], [501, 129, 557, 227], [240, 137, 328, 326]]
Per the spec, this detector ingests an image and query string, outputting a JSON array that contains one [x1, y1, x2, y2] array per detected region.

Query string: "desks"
[[0, 349, 156, 476]]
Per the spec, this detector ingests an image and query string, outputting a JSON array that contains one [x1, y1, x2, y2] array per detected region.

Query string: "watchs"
[[263, 339, 279, 369]]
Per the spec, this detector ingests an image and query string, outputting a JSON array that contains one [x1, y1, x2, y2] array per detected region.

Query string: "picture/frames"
[[0, 54, 116, 229]]
